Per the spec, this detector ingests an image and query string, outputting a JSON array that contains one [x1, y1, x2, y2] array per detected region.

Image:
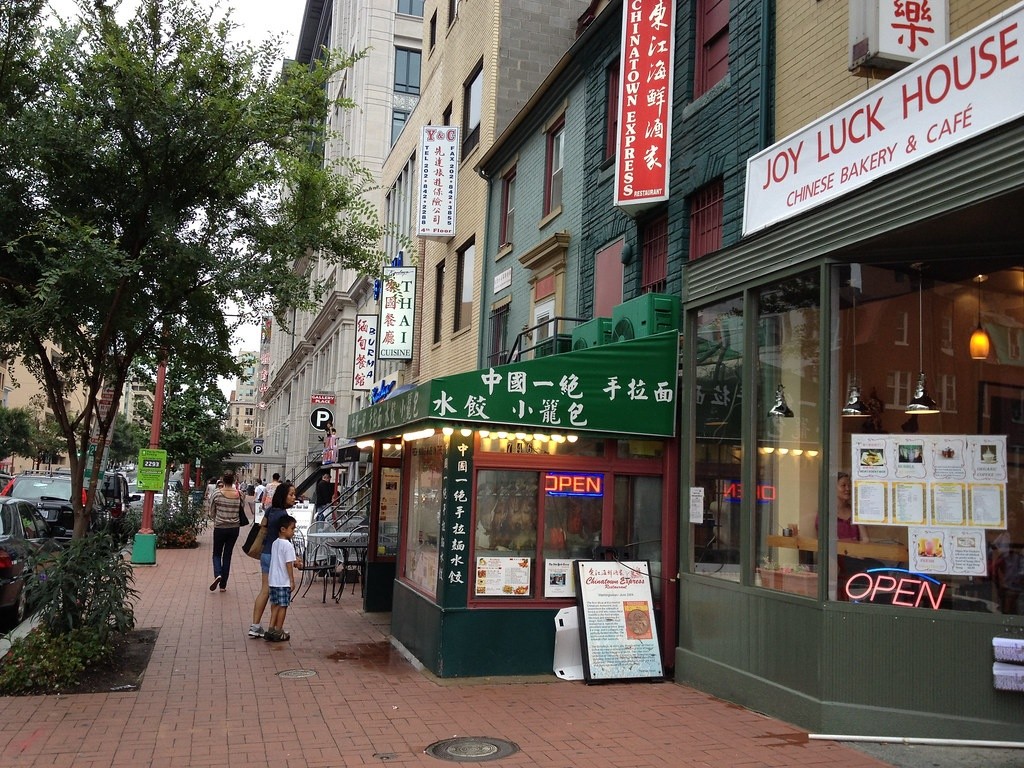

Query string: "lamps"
[[970, 273, 990, 360], [767, 383, 795, 418], [841, 276, 872, 417], [904, 262, 940, 414]]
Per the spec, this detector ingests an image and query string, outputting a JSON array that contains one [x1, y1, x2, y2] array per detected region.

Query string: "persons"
[[264, 515, 297, 642], [204, 478, 219, 518], [234, 478, 292, 495], [247, 483, 297, 639], [253, 479, 265, 504], [989, 507, 1024, 556], [261, 473, 281, 512], [315, 474, 335, 532], [815, 471, 869, 544], [216, 479, 223, 488], [209, 472, 245, 592], [321, 421, 338, 464]]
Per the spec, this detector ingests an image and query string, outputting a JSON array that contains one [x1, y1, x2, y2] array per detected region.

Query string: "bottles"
[[942, 446, 954, 458], [378, 543, 385, 554], [259, 505, 262, 512]]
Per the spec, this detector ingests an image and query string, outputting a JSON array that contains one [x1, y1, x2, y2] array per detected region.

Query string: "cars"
[[0, 463, 195, 550], [0, 495, 67, 627]]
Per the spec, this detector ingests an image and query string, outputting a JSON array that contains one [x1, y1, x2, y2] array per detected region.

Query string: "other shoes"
[[220, 587, 226, 592], [210, 574, 222, 591]]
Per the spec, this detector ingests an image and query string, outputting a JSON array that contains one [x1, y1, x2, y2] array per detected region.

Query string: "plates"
[[861, 452, 883, 465], [959, 538, 974, 546]]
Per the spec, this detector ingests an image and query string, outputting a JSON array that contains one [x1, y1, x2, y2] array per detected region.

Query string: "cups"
[[983, 454, 994, 462], [297, 504, 302, 508], [919, 537, 940, 556], [304, 500, 309, 508], [783, 528, 792, 537], [900, 448, 919, 462]]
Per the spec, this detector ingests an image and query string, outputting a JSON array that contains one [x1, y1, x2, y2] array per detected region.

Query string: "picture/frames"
[[976, 380, 1024, 468]]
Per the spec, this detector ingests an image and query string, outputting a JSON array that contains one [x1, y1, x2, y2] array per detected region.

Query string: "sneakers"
[[249, 623, 265, 637], [273, 631, 290, 641], [264, 631, 275, 640]]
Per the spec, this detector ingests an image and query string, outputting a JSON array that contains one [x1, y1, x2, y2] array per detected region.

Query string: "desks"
[[326, 542, 367, 597], [307, 532, 368, 542], [766, 535, 909, 604]]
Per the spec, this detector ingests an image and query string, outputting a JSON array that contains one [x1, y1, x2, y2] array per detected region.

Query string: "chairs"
[[290, 521, 369, 603]]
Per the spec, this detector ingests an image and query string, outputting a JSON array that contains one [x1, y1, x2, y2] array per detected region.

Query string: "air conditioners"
[[611, 292, 681, 343], [573, 316, 612, 351]]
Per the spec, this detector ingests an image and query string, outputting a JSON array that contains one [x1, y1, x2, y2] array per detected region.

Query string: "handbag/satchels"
[[242, 523, 264, 560], [239, 505, 249, 526]]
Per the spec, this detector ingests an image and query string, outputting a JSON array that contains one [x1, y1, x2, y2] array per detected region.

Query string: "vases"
[[757, 567, 817, 598]]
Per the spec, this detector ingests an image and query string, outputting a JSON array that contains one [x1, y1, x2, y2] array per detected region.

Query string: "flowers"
[[763, 555, 804, 575]]
